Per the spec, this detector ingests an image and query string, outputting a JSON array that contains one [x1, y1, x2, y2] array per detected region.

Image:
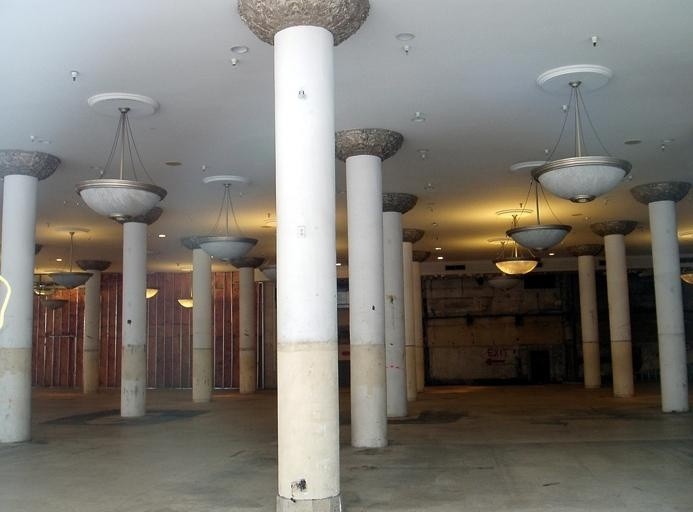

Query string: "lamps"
[[145, 250, 160, 298], [73, 91, 166, 221], [177, 267, 193, 308], [39, 226, 93, 309], [491, 65, 632, 275], [194, 174, 276, 280], [678, 231, 692, 284]]
